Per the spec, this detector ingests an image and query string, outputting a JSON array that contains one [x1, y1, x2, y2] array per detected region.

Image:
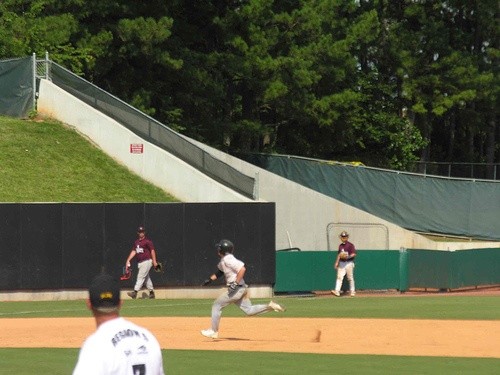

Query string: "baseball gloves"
[[154, 262, 163, 273], [339, 254, 349, 262], [120, 269, 132, 280], [224, 282, 238, 296]]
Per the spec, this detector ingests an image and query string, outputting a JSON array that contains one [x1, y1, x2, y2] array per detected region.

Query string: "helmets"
[[216, 240, 232, 254], [137, 226, 146, 234], [340, 231, 349, 238]]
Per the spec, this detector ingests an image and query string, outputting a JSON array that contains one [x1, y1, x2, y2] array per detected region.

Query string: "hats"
[[90, 274, 120, 308]]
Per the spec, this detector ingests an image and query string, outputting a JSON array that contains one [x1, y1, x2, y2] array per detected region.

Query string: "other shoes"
[[350, 292, 355, 297], [332, 290, 341, 297], [202, 328, 218, 339], [268, 300, 285, 313], [149, 291, 155, 298], [127, 291, 137, 299]]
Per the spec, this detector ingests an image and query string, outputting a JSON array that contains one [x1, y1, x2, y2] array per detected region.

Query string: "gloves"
[[201, 278, 213, 288], [228, 281, 238, 294]]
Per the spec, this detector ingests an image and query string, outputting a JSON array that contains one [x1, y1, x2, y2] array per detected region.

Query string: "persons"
[[72, 274, 164, 375], [198, 239, 285, 339], [331, 230, 357, 297], [126, 227, 158, 299]]
